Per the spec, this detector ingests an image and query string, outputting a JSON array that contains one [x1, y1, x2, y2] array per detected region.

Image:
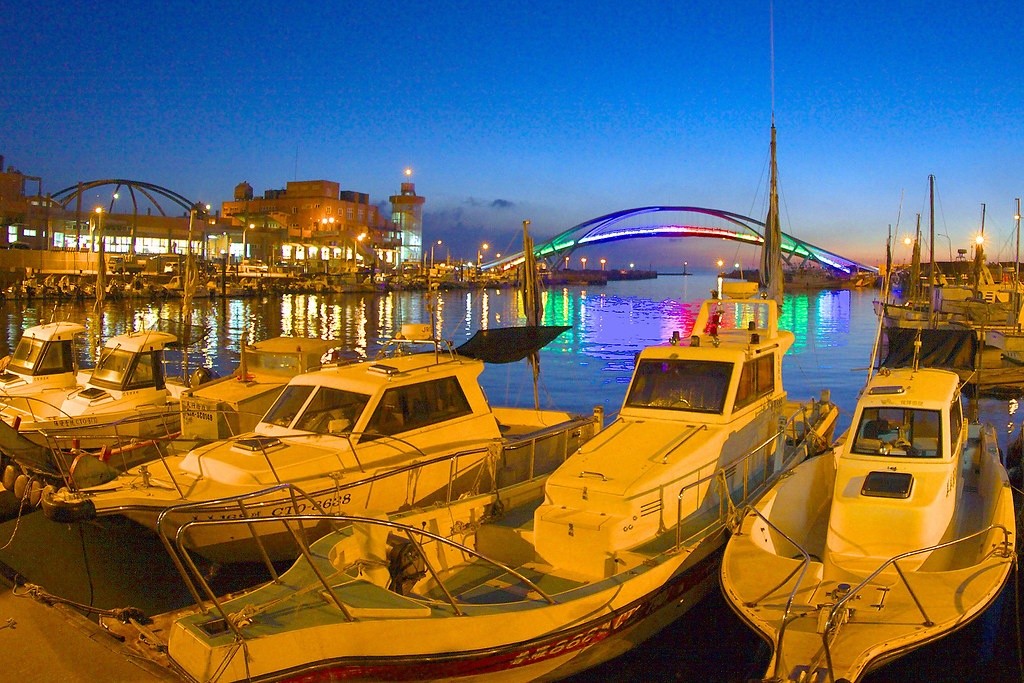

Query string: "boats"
[[40, 205, 607, 572], [158, 26, 841, 682], [718, 188, 1017, 682]]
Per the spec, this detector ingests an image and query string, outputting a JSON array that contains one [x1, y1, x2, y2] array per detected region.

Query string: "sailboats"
[[870, 169, 1023, 400], [0, 181, 564, 478], [709, 109, 786, 311]]
